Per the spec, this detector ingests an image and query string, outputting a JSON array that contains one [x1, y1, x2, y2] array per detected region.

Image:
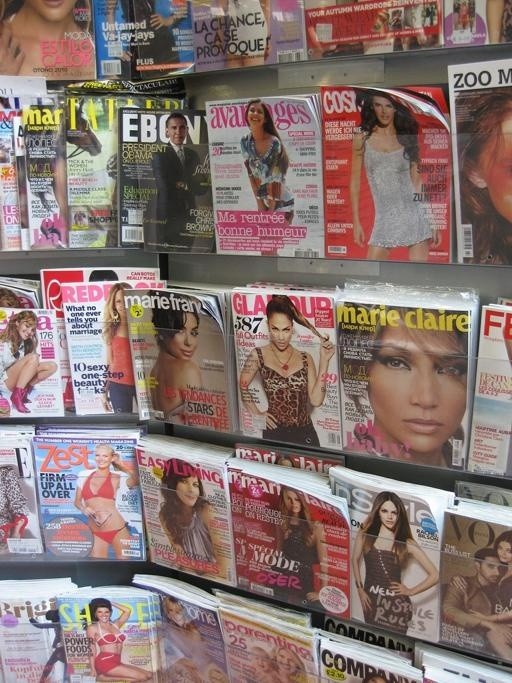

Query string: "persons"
[[0, 466, 28, 551], [0, 0, 96, 80], [351, 491, 440, 636], [239, 295, 335, 446], [267, 486, 328, 612], [486, 1, 511, 43], [158, 458, 227, 582], [338, 302, 468, 471], [217, 0, 271, 69], [74, 444, 141, 561], [145, 113, 208, 253], [100, 281, 140, 414], [106, 0, 188, 80], [87, 598, 153, 683], [161, 596, 228, 683], [239, 98, 294, 257], [147, 290, 205, 424], [251, 647, 279, 683], [442, 548, 509, 656], [351, 89, 441, 263], [0, 310, 58, 414], [459, 89, 512, 265], [30, 609, 69, 683], [468, 530, 512, 665], [271, 646, 306, 683], [445, 1, 487, 46]]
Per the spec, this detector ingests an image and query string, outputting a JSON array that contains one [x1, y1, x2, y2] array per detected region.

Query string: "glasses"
[[91, 507, 113, 528]]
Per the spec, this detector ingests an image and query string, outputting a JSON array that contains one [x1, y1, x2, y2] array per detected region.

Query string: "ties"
[[177, 148, 185, 168]]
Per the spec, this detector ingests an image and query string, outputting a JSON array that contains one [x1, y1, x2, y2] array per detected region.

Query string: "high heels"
[[24, 383, 33, 403], [10, 387, 31, 413]]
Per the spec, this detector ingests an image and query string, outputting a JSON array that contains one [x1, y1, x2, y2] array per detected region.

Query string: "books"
[[1, 0, 512, 683]]
